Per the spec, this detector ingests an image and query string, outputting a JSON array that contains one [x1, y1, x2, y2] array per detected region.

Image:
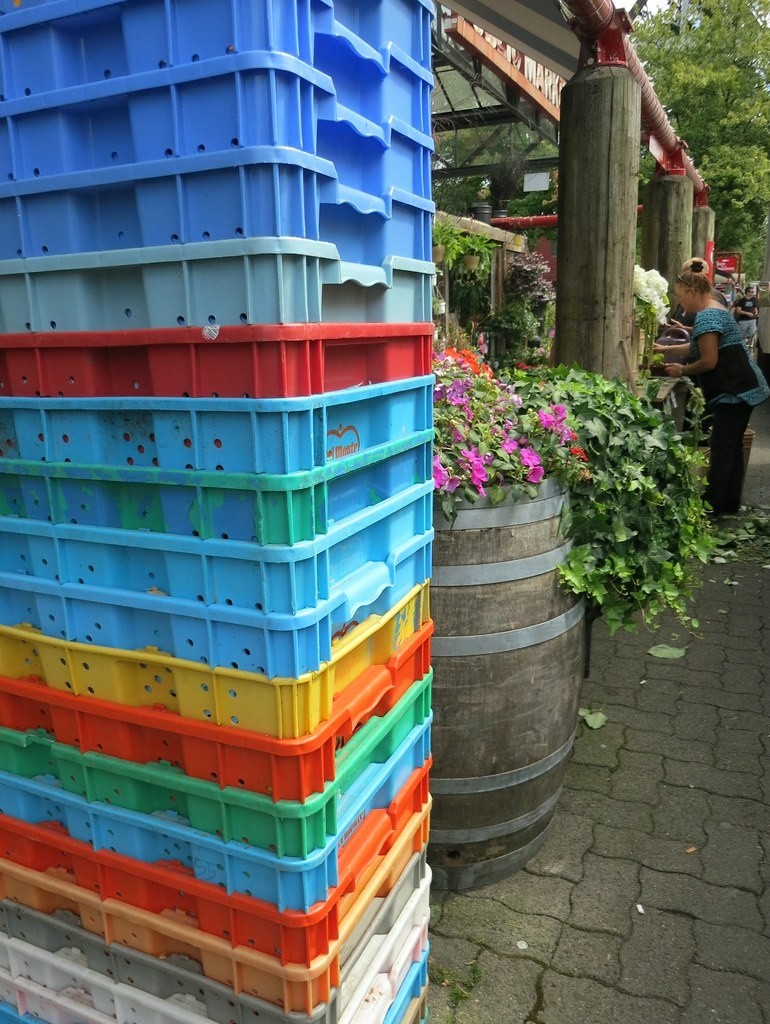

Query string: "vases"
[[426, 476, 588, 890]]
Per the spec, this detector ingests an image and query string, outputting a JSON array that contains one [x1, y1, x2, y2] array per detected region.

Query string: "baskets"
[[707, 426, 756, 492]]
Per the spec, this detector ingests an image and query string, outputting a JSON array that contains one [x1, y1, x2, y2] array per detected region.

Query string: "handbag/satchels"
[[688, 343, 760, 402]]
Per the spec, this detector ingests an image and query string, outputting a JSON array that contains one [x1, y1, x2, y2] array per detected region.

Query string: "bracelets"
[[679, 367, 683, 376], [665, 345, 668, 352]]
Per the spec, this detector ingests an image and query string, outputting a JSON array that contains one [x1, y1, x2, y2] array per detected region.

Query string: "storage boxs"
[[0, 0, 440, 1024]]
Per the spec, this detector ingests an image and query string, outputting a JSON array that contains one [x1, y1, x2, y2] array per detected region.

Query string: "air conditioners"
[[431, 215, 502, 279]]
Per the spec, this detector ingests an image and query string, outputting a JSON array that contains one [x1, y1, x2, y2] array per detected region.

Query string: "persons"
[[651, 258, 769, 515], [736, 286, 758, 354]]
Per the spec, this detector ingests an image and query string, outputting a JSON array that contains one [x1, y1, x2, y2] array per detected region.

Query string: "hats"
[[681, 256, 710, 274]]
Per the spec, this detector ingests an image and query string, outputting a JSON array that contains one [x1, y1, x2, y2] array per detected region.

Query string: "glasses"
[[676, 274, 698, 293]]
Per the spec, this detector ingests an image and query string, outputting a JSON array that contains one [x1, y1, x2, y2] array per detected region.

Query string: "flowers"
[[432, 319, 723, 633]]
